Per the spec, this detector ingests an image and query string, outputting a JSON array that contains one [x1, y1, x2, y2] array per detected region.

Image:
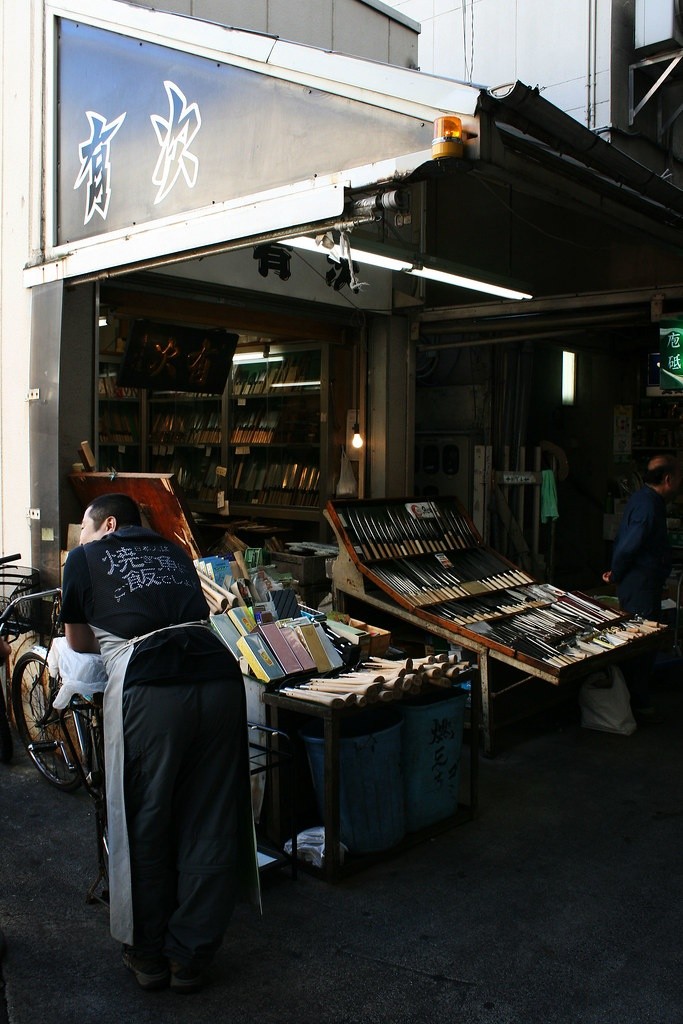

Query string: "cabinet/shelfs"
[[94, 333, 349, 543]]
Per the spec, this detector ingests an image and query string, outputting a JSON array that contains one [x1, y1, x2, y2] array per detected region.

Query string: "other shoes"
[[635, 707, 664, 723]]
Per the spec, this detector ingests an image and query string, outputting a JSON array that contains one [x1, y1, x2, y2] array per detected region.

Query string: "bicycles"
[[1, 553, 109, 909]]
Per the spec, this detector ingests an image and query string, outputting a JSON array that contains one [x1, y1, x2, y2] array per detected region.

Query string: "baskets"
[[0, 564, 42, 633]]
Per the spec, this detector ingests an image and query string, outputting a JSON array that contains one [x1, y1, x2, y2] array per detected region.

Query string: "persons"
[[62, 493, 251, 992], [601, 454, 681, 723]]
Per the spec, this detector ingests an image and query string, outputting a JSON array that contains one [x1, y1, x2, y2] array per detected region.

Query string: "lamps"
[[278, 229, 536, 300]]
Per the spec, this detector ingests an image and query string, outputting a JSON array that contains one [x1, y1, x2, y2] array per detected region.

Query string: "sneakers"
[[122, 950, 171, 989], [169, 959, 211, 992]]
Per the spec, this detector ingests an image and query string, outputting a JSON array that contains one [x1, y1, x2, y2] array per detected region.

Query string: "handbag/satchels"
[[579, 662, 638, 736]]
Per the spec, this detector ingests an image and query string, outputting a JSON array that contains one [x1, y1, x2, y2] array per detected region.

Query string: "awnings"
[[42, 1, 683, 284]]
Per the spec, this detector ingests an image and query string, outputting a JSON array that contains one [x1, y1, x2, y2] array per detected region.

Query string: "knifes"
[[230, 409, 281, 444], [232, 358, 310, 396], [347, 501, 669, 668], [238, 462, 320, 506], [150, 411, 222, 444], [98, 412, 139, 443], [169, 460, 221, 504]]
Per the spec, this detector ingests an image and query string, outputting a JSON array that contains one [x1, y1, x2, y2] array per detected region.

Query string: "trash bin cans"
[[300, 717, 407, 857], [384, 686, 470, 829]]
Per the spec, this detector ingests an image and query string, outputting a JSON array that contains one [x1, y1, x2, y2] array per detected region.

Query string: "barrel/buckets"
[[294, 707, 406, 854], [390, 685, 468, 826]]
